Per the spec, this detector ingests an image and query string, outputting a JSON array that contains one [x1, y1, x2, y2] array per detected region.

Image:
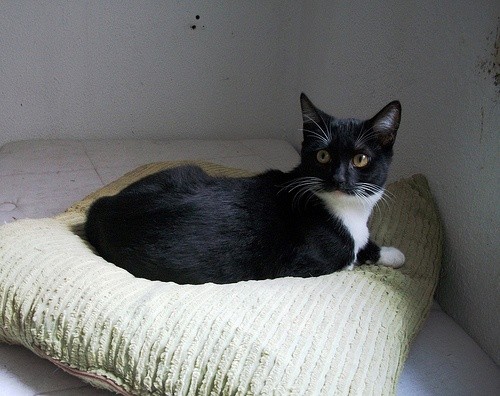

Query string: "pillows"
[[0, 160, 442, 396]]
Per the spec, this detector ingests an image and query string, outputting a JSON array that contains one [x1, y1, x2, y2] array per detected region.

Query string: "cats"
[[82, 91, 405, 285]]
